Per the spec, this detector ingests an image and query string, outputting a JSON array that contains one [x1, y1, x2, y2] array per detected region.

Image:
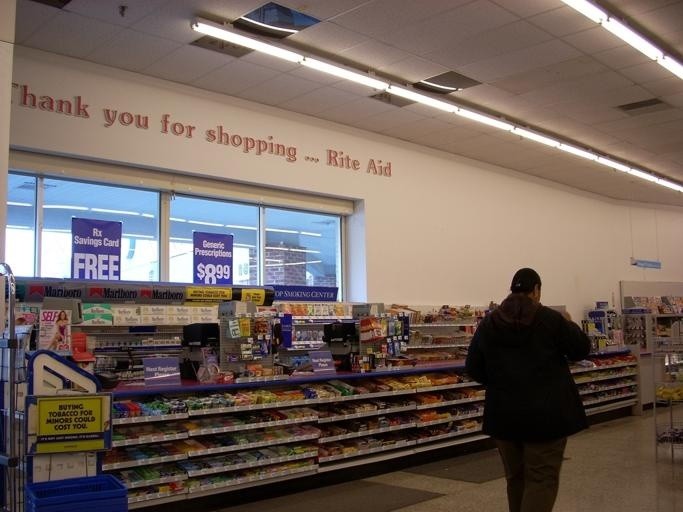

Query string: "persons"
[[465, 267, 592, 512], [46, 311, 69, 352]]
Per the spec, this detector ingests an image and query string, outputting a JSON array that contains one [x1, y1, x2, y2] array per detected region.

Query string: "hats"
[[509, 268, 541, 294]]
